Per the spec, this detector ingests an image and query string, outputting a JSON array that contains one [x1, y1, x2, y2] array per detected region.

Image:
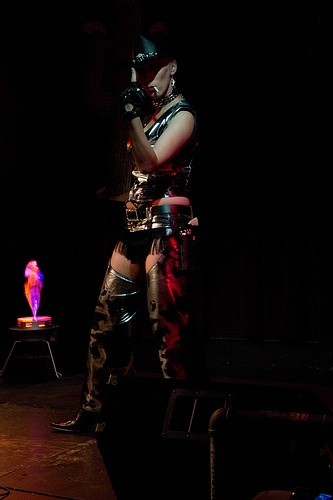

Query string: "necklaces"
[[152, 89, 180, 107]]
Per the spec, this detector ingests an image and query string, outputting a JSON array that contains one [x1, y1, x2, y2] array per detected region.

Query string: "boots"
[[145, 264, 192, 380], [49, 264, 141, 434]]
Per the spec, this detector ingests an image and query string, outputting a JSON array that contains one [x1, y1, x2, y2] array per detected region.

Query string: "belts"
[[127, 205, 191, 222]]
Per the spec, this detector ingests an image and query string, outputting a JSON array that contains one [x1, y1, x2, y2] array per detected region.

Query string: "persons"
[[49, 33, 198, 435]]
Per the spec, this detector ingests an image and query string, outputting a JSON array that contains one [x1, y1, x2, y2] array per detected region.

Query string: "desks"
[[207, 375, 333, 500], [0, 323, 64, 384]]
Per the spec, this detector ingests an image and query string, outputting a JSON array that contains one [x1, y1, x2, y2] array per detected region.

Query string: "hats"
[[129, 22, 177, 67]]
